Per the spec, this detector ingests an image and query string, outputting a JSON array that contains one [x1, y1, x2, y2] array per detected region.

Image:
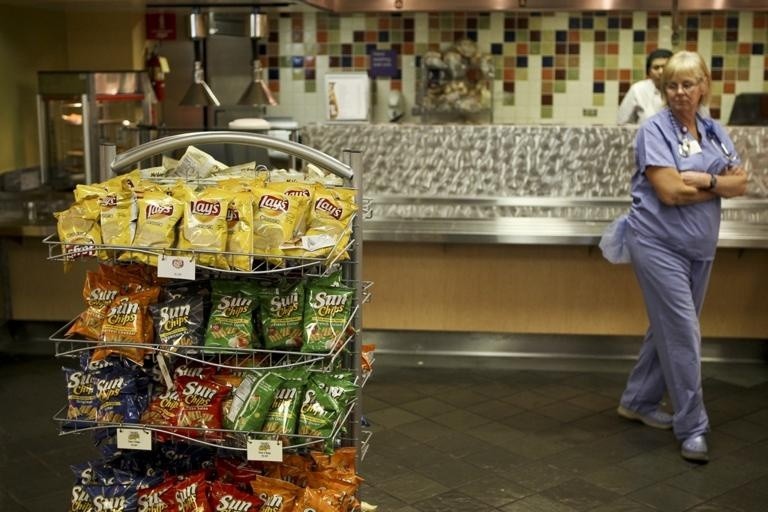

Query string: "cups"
[[667, 83, 694, 93]]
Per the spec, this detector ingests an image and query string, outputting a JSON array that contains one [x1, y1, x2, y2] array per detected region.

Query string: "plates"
[[45, 132, 376, 512]]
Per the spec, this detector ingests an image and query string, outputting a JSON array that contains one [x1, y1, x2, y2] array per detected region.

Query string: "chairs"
[[682, 435, 709, 461], [616, 405, 673, 430]]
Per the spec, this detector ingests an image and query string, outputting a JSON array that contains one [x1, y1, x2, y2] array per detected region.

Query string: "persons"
[[617, 48, 748, 462], [617, 49, 710, 125]]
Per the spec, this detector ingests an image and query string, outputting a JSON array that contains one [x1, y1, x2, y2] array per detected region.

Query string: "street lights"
[[599, 214, 633, 264]]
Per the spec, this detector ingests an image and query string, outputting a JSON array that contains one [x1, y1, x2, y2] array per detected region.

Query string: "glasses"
[[180, 2, 276, 109]]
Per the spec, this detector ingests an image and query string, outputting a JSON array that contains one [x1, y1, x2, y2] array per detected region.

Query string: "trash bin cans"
[[702, 172, 717, 191]]
